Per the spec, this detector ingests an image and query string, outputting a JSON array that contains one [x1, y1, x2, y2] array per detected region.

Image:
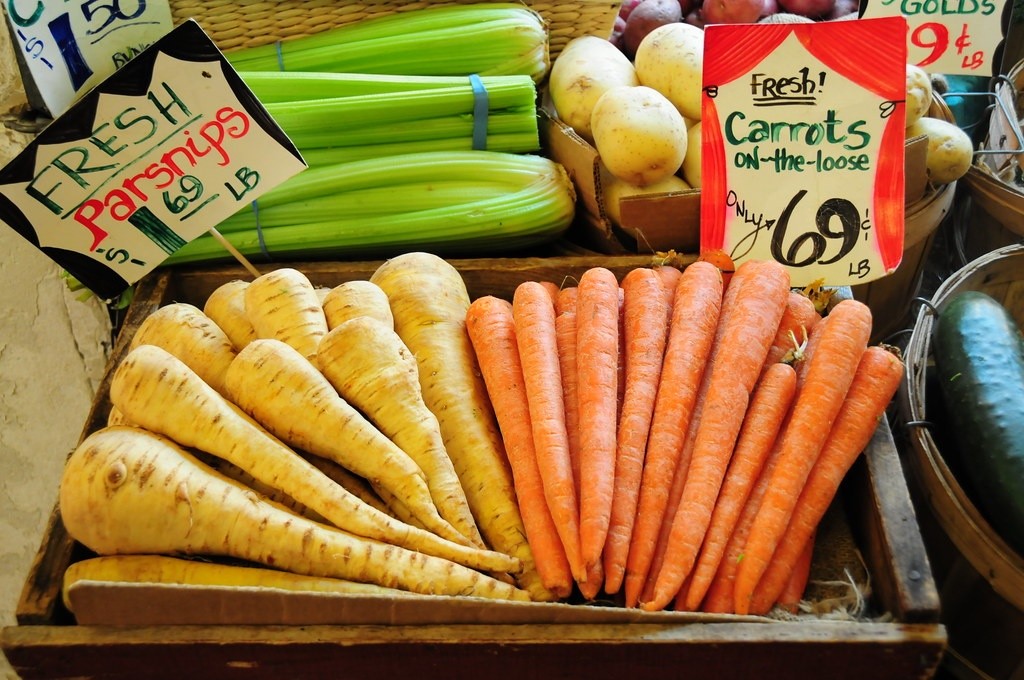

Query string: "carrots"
[[466, 249, 902, 616]]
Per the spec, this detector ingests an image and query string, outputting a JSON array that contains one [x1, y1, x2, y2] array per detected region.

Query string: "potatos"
[[547, 0, 973, 231]]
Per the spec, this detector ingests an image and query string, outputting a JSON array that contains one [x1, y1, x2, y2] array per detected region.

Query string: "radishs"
[[62, 250, 553, 612]]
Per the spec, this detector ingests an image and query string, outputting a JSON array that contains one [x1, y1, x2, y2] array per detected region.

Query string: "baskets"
[[171, 0, 621, 67]]
